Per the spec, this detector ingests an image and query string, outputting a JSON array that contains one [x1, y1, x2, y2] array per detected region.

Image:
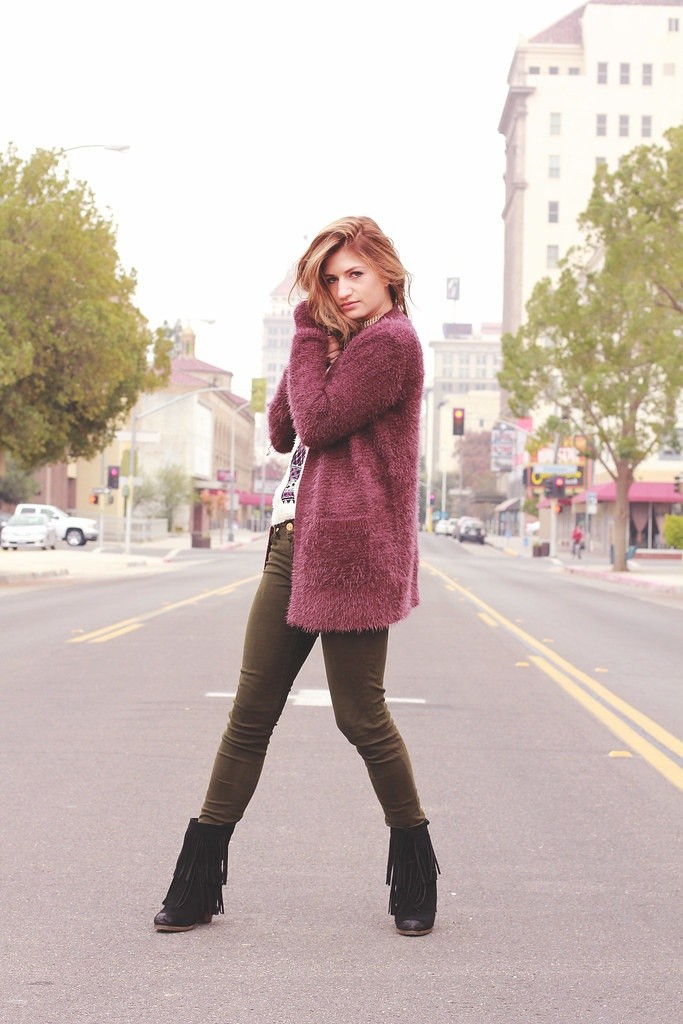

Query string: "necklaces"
[[360, 303, 393, 328]]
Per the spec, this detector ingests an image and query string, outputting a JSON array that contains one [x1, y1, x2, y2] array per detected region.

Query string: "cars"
[[446, 518, 459, 536], [435, 520, 446, 534], [0, 513, 56, 550]]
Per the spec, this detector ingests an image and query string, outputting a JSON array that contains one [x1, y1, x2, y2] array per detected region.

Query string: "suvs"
[[452, 515, 485, 544], [13, 504, 98, 548]]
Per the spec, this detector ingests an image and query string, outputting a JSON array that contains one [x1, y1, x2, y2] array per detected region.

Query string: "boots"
[[386, 819, 440, 936], [154, 818, 235, 933]]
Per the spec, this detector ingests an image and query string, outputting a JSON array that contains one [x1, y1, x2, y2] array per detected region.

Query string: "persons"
[[154, 217, 440, 937], [572, 525, 585, 554]]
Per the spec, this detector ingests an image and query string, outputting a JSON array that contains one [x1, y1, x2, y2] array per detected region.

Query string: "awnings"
[[201, 489, 274, 505], [495, 497, 520, 511], [537, 497, 571, 508], [572, 483, 683, 503]]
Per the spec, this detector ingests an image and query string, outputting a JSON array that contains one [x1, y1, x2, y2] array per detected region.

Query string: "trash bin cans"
[[540, 542, 549, 556], [192, 532, 202, 548]]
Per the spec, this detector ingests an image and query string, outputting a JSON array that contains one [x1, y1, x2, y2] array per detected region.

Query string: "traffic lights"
[[90, 495, 98, 503], [431, 495, 436, 505], [123, 387, 235, 552], [453, 408, 465, 436], [108, 466, 119, 489]]
